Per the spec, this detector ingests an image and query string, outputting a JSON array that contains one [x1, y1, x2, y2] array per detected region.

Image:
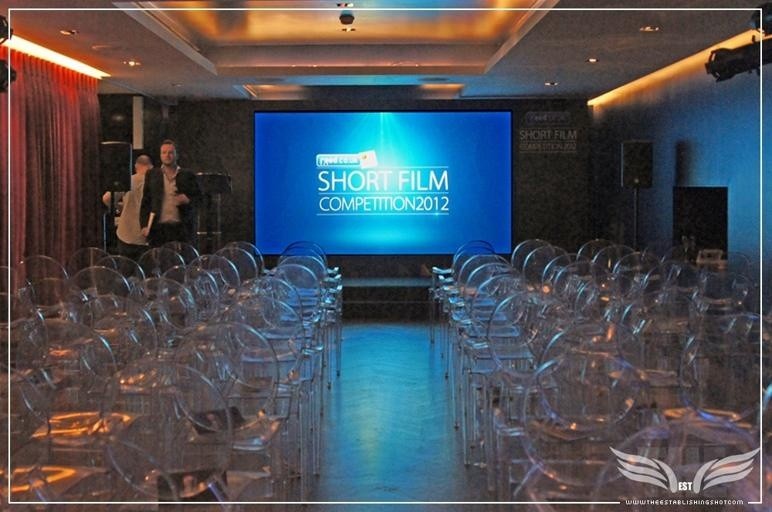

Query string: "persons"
[[98, 152, 164, 269], [138, 137, 201, 254]]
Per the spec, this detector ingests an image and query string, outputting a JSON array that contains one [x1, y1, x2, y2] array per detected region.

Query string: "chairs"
[[0, 238, 344, 512], [428, 239, 772, 512]]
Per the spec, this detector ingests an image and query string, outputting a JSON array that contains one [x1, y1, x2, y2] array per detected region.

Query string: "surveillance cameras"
[[340, 11, 355, 24]]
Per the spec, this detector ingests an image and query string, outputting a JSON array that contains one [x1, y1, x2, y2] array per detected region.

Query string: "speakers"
[[620, 139, 654, 190], [98, 141, 132, 193]]
